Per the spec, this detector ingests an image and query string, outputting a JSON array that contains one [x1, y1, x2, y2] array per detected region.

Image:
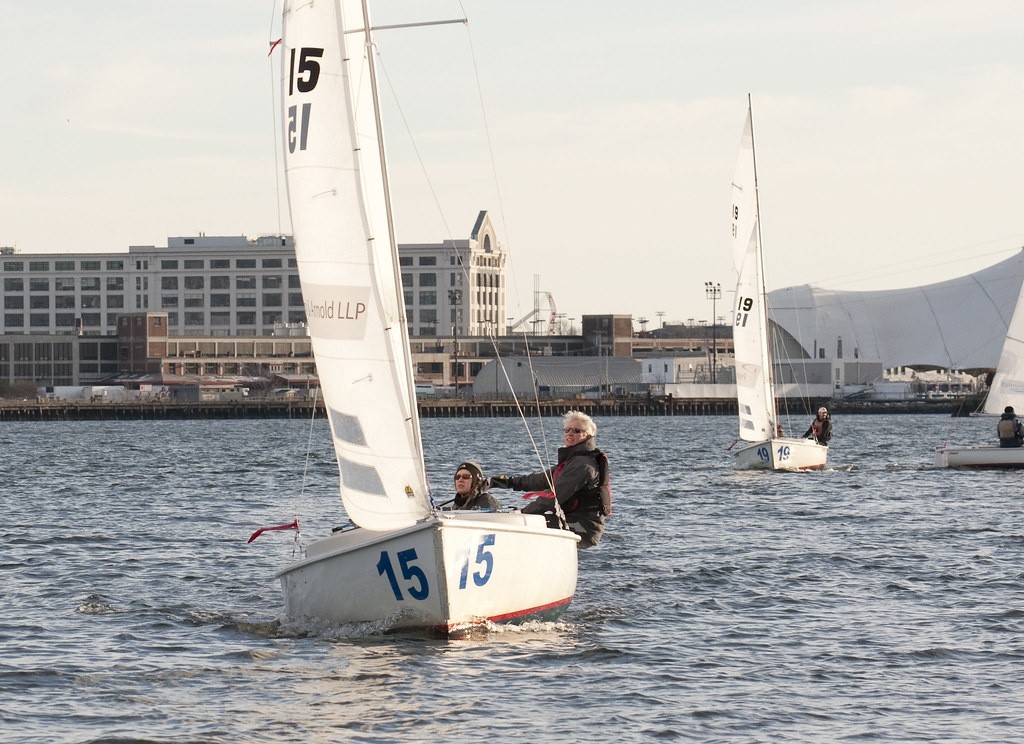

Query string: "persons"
[[451, 462, 503, 513], [773, 425, 784, 438], [997, 406, 1024, 447], [801, 407, 833, 447], [484, 410, 608, 550]]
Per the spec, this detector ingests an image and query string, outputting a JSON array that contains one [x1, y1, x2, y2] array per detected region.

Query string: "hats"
[[456, 460, 483, 490], [818, 407, 827, 413]]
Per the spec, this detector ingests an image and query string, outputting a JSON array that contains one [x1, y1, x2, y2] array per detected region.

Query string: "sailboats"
[[264, 1, 580, 643], [730, 93, 830, 472]]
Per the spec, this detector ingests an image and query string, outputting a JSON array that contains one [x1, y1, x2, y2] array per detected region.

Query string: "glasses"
[[563, 427, 588, 434], [777, 431, 783, 433], [454, 474, 473, 480]]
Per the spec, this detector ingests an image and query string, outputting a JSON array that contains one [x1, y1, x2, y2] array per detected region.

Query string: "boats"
[[942, 445, 1024, 470]]
